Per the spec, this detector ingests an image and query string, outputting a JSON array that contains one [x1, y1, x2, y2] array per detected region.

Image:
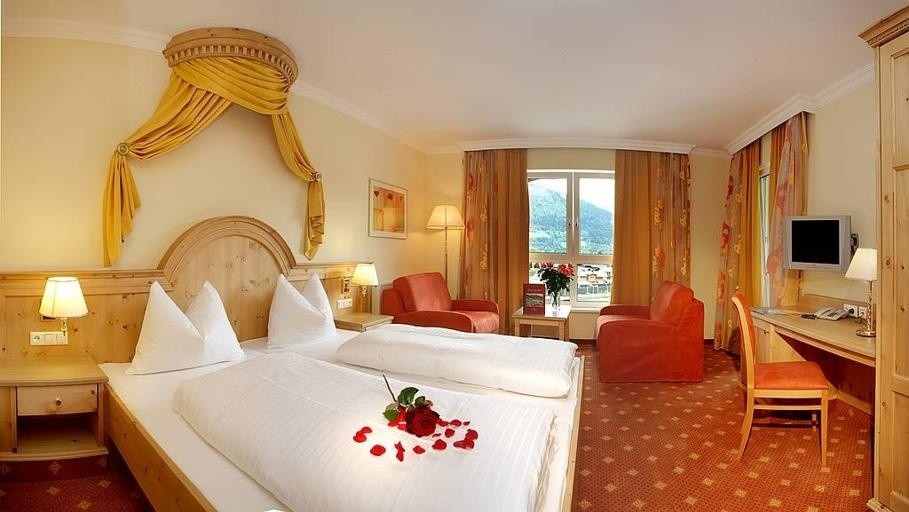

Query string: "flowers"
[[538, 258, 575, 306], [376, 369, 438, 440]]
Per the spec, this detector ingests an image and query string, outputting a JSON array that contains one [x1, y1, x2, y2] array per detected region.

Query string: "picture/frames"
[[368, 177, 409, 240]]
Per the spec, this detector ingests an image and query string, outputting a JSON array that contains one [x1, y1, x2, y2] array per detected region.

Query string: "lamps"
[[40, 275, 87, 338], [425, 203, 467, 286], [844, 248, 879, 337], [341, 262, 378, 298]]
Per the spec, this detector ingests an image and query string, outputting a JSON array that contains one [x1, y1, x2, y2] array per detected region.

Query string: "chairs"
[[595, 280, 705, 386], [381, 271, 500, 333], [729, 290, 831, 470]]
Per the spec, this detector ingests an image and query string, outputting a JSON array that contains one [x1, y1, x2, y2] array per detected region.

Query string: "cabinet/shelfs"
[[855, 0, 909, 512]]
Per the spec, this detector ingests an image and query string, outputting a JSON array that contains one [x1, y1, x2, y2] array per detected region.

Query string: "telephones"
[[814, 307, 849, 321]]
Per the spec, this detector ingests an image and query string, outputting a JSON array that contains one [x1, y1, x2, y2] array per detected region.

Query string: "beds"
[[97, 215, 587, 512]]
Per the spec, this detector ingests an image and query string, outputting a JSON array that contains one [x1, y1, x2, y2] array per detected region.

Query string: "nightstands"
[[0, 357, 111, 484], [333, 312, 394, 333]]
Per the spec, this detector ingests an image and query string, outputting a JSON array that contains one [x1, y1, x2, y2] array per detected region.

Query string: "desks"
[[740, 294, 876, 412], [512, 303, 572, 341]]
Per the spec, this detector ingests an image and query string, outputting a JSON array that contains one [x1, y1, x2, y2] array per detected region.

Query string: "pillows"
[[266, 271, 337, 349], [126, 278, 243, 378]]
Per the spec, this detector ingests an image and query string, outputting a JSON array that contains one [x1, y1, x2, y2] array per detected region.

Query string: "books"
[[523, 284, 545, 314]]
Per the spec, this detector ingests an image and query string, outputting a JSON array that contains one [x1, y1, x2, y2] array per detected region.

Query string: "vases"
[[549, 291, 562, 309]]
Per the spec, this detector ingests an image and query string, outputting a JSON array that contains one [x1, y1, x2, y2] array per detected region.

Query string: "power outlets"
[[843, 303, 858, 318]]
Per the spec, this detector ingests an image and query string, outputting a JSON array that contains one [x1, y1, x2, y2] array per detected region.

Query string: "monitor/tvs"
[[784, 215, 854, 271]]
